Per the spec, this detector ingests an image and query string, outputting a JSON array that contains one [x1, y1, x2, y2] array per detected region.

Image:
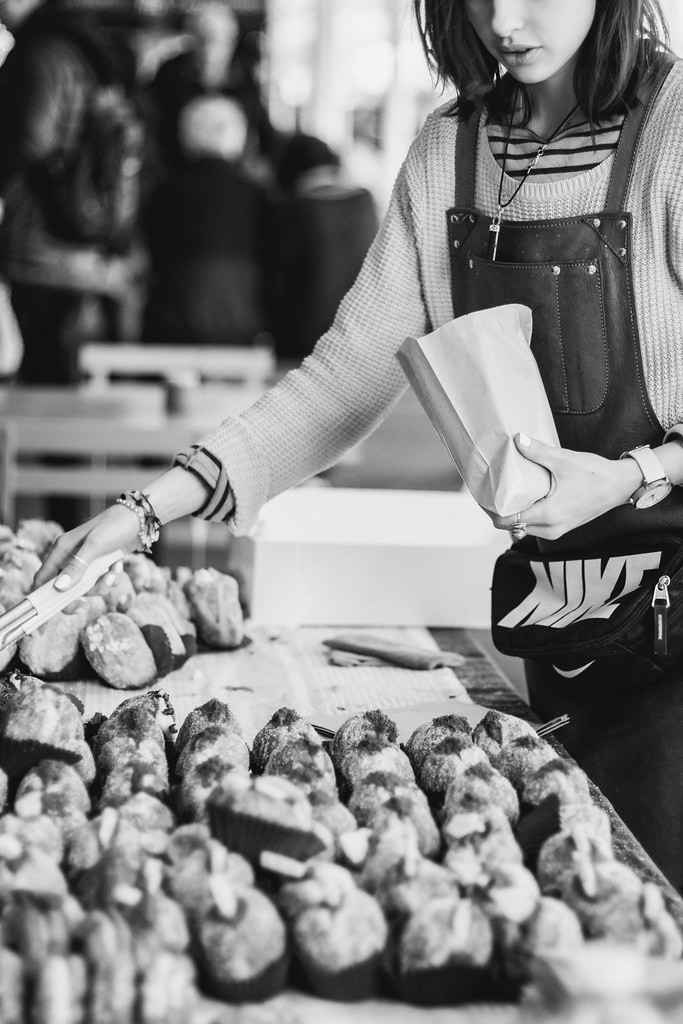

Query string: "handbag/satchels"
[[490, 528, 683, 671]]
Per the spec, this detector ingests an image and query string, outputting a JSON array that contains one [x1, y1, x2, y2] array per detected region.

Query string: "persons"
[[34, 0, 683, 898], [16, 1, 379, 358]]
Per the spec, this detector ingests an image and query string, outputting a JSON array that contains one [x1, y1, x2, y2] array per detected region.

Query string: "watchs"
[[619, 445, 673, 510]]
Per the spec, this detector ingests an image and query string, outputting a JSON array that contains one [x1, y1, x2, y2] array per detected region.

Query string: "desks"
[[45, 625, 683, 1024]]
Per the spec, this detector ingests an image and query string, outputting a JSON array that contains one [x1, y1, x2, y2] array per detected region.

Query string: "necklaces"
[[489, 89, 578, 262]]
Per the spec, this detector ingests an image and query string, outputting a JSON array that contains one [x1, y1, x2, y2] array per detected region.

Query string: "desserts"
[[0, 516, 683, 1024]]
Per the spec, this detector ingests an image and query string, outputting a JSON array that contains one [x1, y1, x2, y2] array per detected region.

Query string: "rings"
[[512, 513, 527, 539], [72, 555, 87, 567]]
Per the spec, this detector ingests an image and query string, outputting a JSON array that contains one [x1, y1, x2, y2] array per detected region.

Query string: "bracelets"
[[116, 491, 162, 554]]
